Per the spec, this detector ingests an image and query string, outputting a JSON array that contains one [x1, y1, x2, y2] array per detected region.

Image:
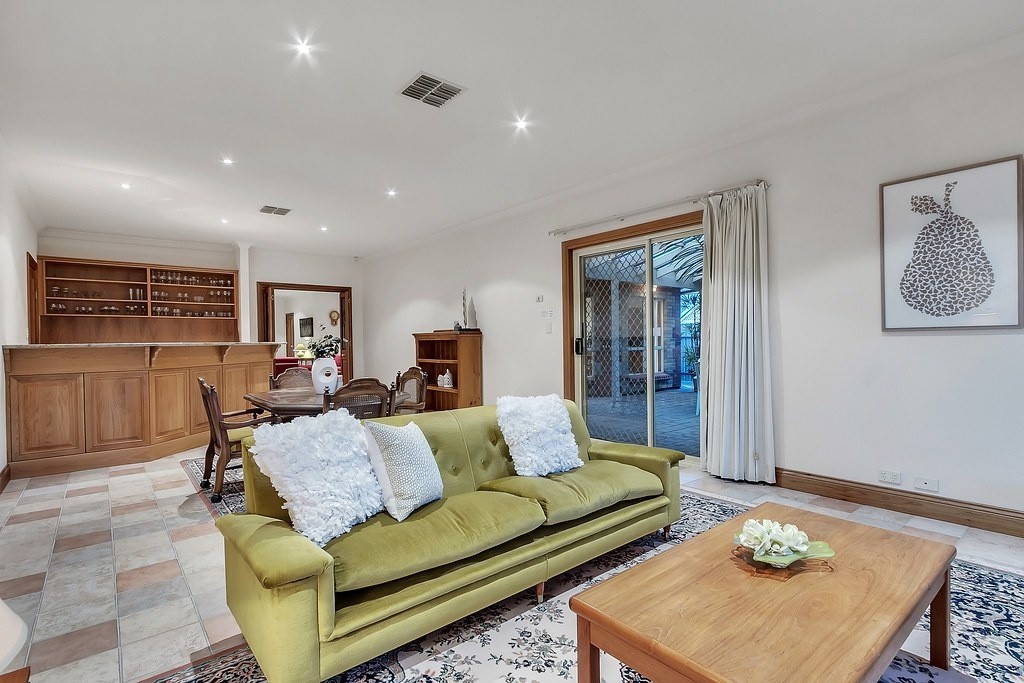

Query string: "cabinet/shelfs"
[[37, 255, 239, 319], [412, 331, 483, 414]]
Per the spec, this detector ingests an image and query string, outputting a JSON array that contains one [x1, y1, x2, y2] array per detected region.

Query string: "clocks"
[[329, 311, 339, 327]]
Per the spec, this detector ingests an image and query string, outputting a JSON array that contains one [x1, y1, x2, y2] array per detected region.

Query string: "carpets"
[[181, 456, 246, 519], [135, 488, 1024, 683]]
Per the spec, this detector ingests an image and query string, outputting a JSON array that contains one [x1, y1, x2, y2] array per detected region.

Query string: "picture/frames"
[[299, 317, 314, 337], [879, 154, 1024, 332]]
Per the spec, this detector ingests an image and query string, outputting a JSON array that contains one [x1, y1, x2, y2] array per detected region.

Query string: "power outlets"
[[877, 469, 901, 485], [913, 475, 939, 492]]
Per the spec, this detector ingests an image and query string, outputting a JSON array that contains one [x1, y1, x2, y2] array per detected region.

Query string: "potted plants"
[[306, 321, 350, 395], [683, 346, 698, 392]]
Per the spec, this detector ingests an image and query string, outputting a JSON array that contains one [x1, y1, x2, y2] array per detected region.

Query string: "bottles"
[[128, 286, 143, 300], [443, 368, 454, 388], [438, 374, 444, 386]]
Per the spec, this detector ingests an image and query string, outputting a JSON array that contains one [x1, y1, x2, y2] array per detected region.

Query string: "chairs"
[[323, 377, 397, 420], [394, 366, 429, 415], [196, 376, 277, 503], [268, 367, 318, 424], [693, 361, 700, 416]]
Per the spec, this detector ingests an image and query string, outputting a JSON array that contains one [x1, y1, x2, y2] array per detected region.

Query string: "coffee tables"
[[569, 501, 956, 683]]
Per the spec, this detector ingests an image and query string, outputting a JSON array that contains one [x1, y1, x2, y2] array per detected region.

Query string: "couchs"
[[273, 354, 341, 379], [215, 393, 686, 683]]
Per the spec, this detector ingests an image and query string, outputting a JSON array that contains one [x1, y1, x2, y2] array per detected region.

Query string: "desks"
[[243, 386, 425, 426]]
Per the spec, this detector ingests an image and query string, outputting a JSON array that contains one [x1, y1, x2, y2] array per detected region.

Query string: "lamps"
[[293, 344, 308, 357]]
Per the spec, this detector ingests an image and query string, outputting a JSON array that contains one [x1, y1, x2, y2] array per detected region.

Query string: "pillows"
[[363, 419, 444, 523], [494, 393, 584, 477], [246, 407, 385, 548]]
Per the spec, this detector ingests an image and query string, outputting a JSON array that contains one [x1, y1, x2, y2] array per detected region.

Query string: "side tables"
[[297, 358, 314, 365]]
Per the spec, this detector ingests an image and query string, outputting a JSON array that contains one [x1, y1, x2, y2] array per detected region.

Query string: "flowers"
[[739, 518, 810, 556]]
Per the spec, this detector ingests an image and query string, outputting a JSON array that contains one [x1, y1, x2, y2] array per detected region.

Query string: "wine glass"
[[50, 286, 101, 299], [50, 304, 148, 315], [151, 289, 232, 302], [151, 271, 234, 288], [151, 306, 232, 317]]
